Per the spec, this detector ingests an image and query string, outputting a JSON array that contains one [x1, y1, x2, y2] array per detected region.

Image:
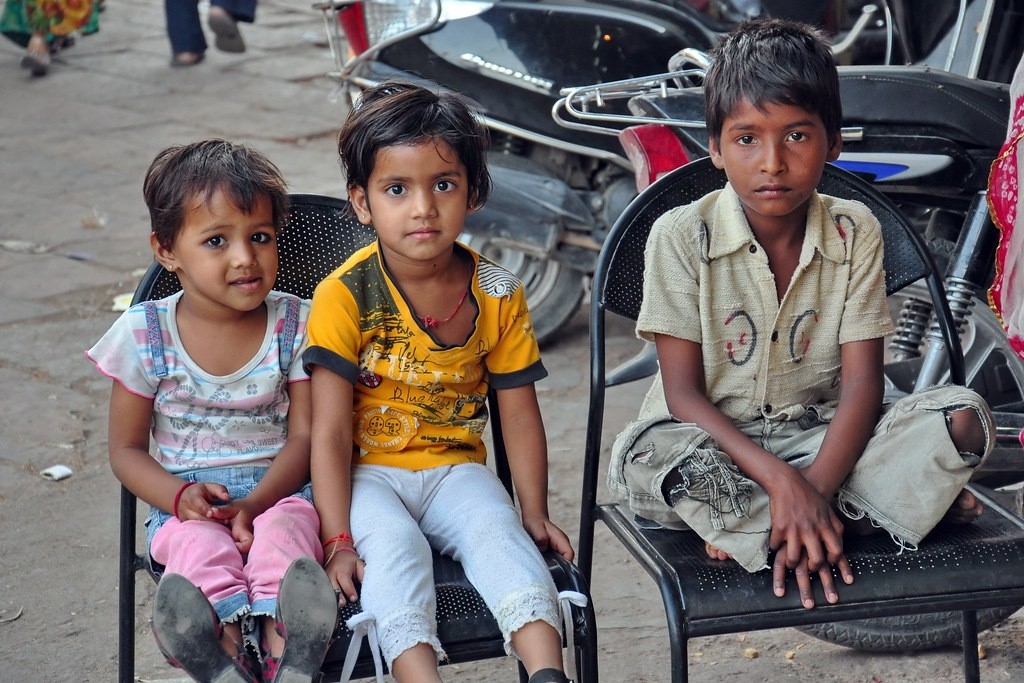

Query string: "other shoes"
[[19, 53, 49, 78], [205, 8, 246, 53], [173, 52, 204, 66], [48, 35, 75, 56], [149, 573, 255, 683], [260, 556, 337, 683]]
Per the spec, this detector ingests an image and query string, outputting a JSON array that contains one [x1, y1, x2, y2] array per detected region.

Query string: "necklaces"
[[407, 279, 478, 327]]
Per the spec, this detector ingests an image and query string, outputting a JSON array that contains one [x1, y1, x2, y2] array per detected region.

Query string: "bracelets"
[[174, 482, 197, 519], [319, 530, 359, 568]]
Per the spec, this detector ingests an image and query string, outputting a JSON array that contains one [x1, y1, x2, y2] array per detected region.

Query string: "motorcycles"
[[312, 0, 1024, 493]]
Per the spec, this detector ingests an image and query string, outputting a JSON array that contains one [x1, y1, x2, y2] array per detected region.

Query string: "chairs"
[[577, 156, 1024, 683], [118, 194, 597, 683]]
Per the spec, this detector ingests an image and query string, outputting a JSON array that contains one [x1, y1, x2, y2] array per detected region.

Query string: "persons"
[[610, 29, 997, 610], [163, 1, 254, 68], [88, 142, 336, 683], [3, 2, 103, 74], [301, 82, 574, 682]]
